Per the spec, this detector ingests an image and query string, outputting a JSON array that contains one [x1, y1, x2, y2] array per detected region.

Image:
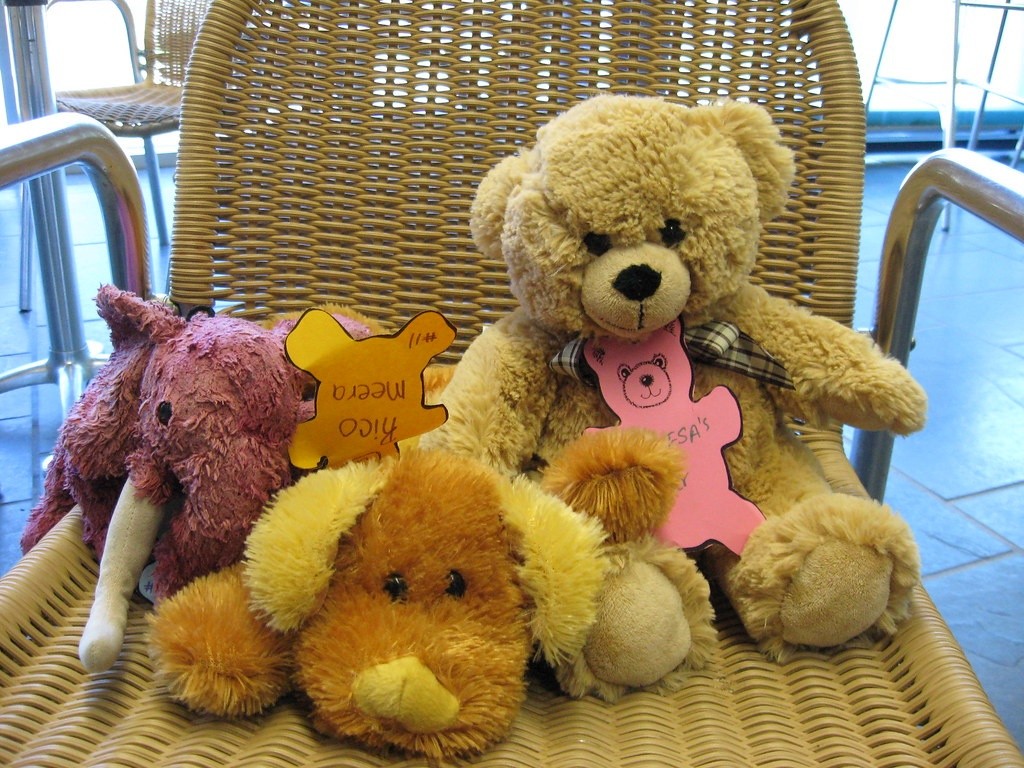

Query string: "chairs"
[[2, 0, 1024, 768], [17, 2, 211, 313]]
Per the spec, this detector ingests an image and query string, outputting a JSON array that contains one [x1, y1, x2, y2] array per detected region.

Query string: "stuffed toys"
[[21, 284, 372, 604], [79, 477, 164, 673], [144, 303, 612, 762], [417, 95, 926, 703]]
